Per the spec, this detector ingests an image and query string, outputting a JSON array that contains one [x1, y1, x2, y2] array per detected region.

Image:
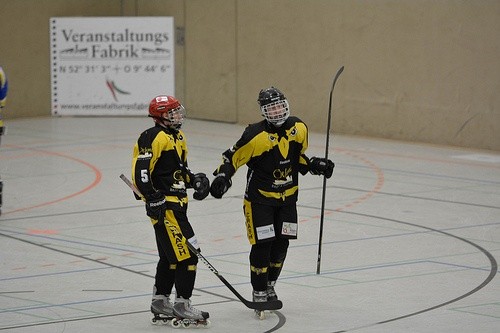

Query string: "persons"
[[0, 67, 9, 215], [131, 95, 211, 328], [210, 86, 335, 321]]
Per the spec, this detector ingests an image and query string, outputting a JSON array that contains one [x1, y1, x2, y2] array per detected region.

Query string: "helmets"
[[148, 95, 184, 128], [257, 85, 290, 125]]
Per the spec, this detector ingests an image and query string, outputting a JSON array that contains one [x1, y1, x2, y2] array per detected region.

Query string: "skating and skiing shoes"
[[150, 295, 174, 326], [251, 290, 268, 320], [170, 298, 210, 328], [268, 281, 280, 312]]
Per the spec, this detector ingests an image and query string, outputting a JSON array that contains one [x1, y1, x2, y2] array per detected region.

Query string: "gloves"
[[208, 174, 232, 198], [144, 190, 167, 220], [190, 172, 209, 200], [307, 156, 336, 180]]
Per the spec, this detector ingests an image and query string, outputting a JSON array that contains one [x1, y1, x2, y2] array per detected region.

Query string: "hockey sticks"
[[310, 64, 348, 278], [115, 173, 288, 310]]
